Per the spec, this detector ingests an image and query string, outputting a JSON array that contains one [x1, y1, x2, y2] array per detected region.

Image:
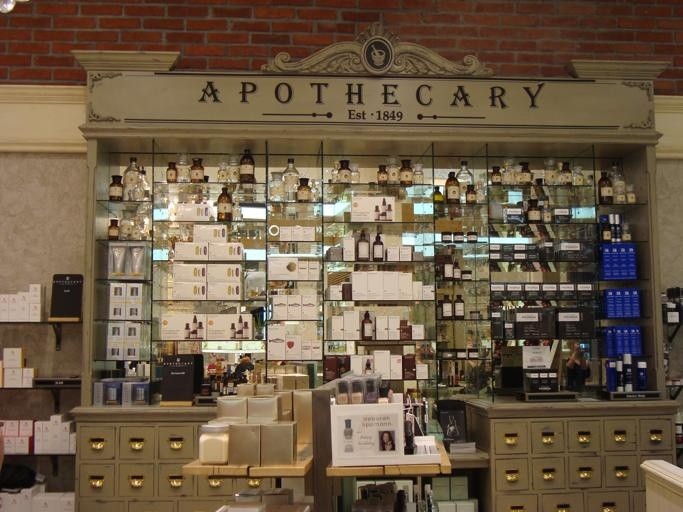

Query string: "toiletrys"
[[607, 360, 616, 392], [637, 360, 647, 390], [197, 371, 248, 397], [344, 418, 353, 440], [614, 213, 621, 242], [608, 213, 615, 243], [332, 376, 395, 407], [622, 354, 633, 392], [615, 360, 624, 392]]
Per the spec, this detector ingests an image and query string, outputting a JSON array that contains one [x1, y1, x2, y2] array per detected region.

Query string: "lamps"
[[0, 0, 31, 15]]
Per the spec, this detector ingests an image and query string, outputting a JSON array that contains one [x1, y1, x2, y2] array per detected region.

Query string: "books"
[[441, 436, 478, 454]]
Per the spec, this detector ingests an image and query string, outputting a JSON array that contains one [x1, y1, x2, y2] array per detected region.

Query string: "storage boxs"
[[196, 383, 314, 511]]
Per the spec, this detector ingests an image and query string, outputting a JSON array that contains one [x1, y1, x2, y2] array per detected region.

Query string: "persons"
[[379, 430, 394, 451], [444, 412, 459, 435], [234, 353, 254, 377], [563, 339, 591, 393]]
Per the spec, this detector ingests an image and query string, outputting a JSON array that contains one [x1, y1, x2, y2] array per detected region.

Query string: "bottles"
[[228, 156, 240, 182], [374, 198, 391, 221], [184, 315, 203, 340], [217, 186, 231, 220], [206, 373, 247, 396], [604, 353, 647, 393], [270, 157, 319, 202], [190, 157, 204, 182], [240, 150, 254, 182], [596, 163, 636, 203], [437, 292, 464, 320], [356, 230, 370, 261], [176, 155, 190, 181], [229, 317, 249, 339], [373, 234, 383, 261], [491, 157, 592, 185], [435, 244, 459, 280], [601, 212, 632, 241], [434, 157, 485, 203], [330, 156, 428, 184], [217, 161, 228, 181], [361, 311, 373, 340], [165, 162, 177, 181], [109, 219, 120, 239], [108, 157, 150, 201]]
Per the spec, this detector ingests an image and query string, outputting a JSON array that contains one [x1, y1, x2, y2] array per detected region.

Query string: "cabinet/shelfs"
[[466, 398, 680, 512], [0, 318, 81, 478], [69, 404, 280, 511]]
